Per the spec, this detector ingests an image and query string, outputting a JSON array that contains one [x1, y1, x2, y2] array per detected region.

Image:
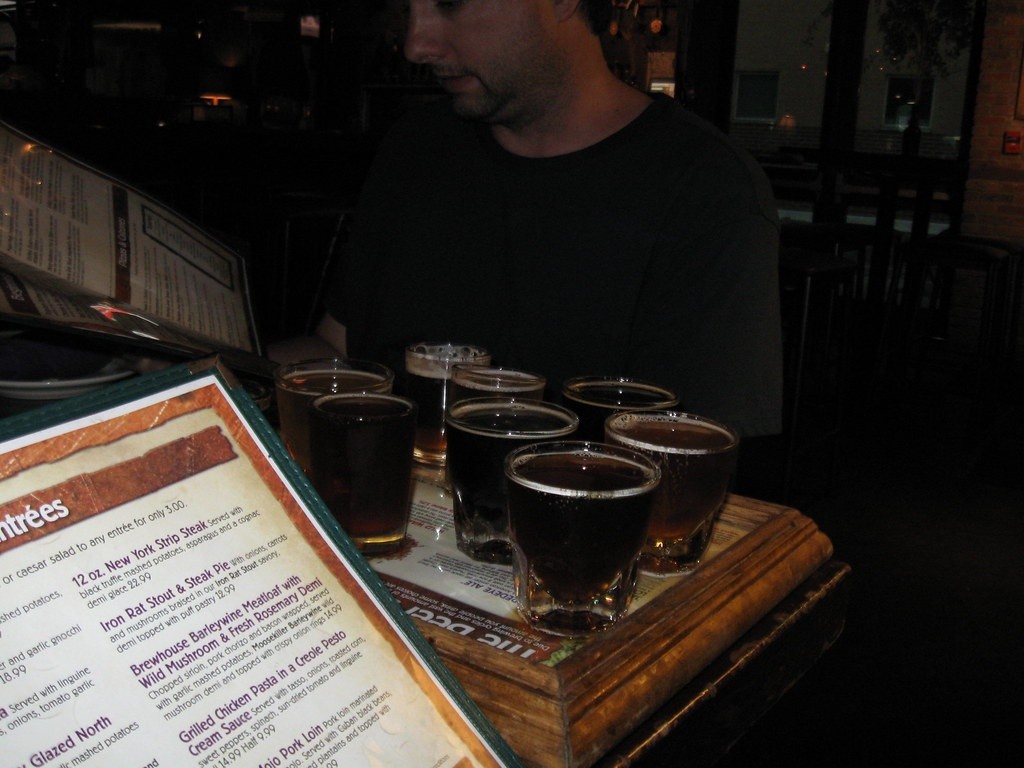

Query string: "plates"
[[0, 330, 136, 399]]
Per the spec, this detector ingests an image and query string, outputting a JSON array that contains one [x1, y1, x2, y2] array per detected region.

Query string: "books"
[[0, 120, 283, 381], [0, 357, 525, 768]]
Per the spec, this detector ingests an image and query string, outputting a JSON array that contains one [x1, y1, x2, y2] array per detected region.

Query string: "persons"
[[124, 0, 783, 441]]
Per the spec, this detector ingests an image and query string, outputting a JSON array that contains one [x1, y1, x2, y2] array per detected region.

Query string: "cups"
[[445, 365, 546, 498], [444, 397, 580, 567], [503, 440, 662, 638], [307, 392, 418, 554], [561, 377, 681, 444], [404, 341, 492, 467], [273, 356, 396, 478], [604, 411, 738, 578]]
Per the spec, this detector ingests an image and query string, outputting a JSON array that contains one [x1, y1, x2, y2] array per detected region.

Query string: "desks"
[[344, 421, 851, 768]]
